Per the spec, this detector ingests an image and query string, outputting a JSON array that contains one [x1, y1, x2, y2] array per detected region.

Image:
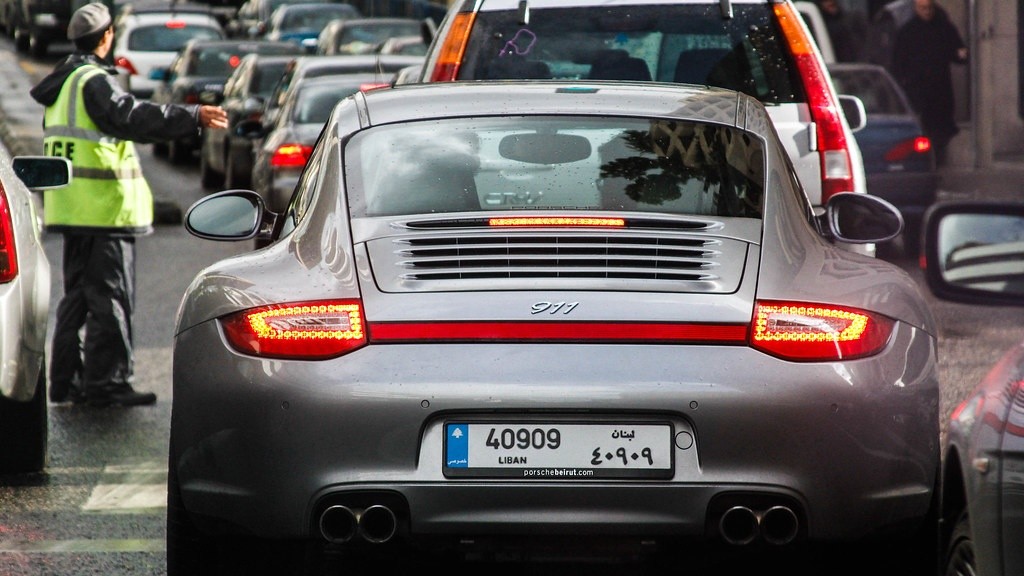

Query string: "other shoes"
[[49, 381, 84, 404], [115, 387, 158, 407]]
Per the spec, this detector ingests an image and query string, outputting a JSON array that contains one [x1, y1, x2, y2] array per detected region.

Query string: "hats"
[[65, 1, 113, 40]]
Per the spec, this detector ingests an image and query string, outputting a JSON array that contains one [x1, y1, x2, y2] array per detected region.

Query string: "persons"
[[31, 3, 229, 410], [896, 1, 971, 169]]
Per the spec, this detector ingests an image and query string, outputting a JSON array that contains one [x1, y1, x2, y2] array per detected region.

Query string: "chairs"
[[371, 131, 758, 221], [488, 45, 747, 100]]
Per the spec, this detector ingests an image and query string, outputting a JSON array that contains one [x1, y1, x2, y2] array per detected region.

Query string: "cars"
[[0, 0, 449, 256], [166, 80, 945, 576], [392, 0, 879, 259], [824, 61, 940, 256], [0, 140, 73, 479], [925, 199, 1023, 575]]
